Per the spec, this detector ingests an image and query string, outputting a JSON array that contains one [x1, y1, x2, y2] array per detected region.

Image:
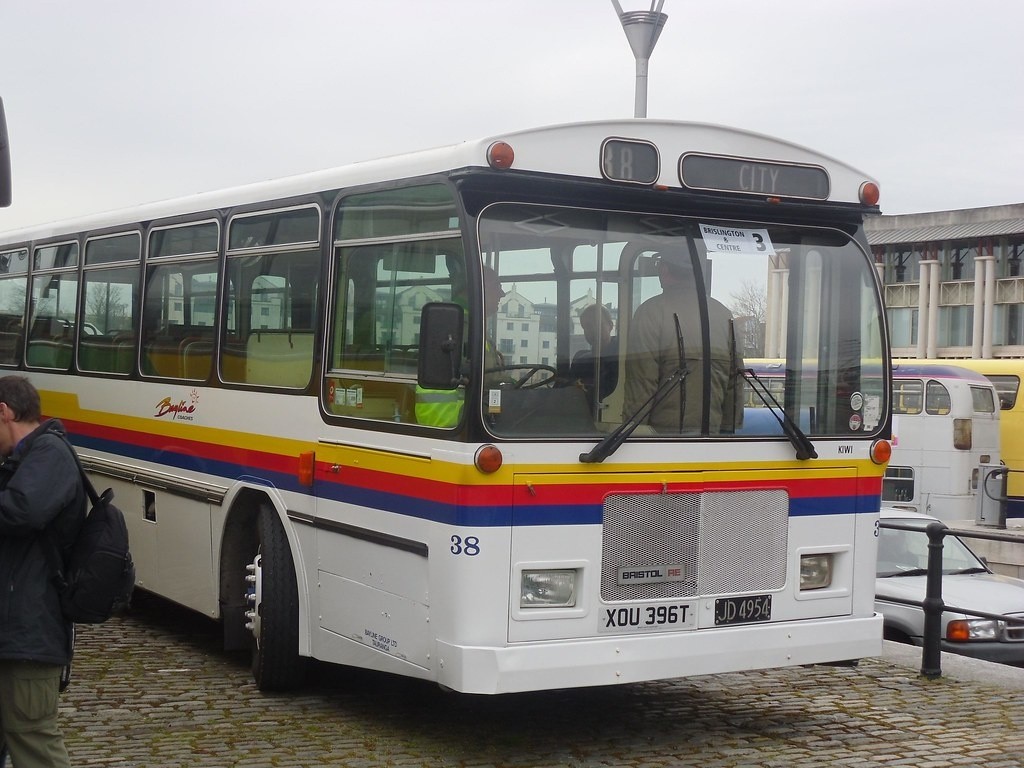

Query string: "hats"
[[651, 242, 707, 268]]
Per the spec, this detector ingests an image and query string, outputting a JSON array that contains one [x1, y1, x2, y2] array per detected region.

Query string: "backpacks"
[[17, 428, 135, 624]]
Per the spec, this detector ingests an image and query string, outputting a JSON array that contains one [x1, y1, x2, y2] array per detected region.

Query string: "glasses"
[[653, 258, 663, 270]]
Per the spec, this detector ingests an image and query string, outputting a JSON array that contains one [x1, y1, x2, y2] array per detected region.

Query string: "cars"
[[875, 507, 1024, 667]]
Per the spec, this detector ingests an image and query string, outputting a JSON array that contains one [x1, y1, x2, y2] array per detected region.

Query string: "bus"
[[891, 359, 1024, 518], [0, 117, 895, 693], [743, 362, 1000, 529]]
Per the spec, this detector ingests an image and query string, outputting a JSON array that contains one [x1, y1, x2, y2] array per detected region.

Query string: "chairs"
[[0, 313, 520, 384]]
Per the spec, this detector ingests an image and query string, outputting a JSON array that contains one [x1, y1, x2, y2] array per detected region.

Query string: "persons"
[[619, 238, 745, 438], [415, 266, 512, 429], [553, 304, 619, 415], [0, 374, 86, 768]]
